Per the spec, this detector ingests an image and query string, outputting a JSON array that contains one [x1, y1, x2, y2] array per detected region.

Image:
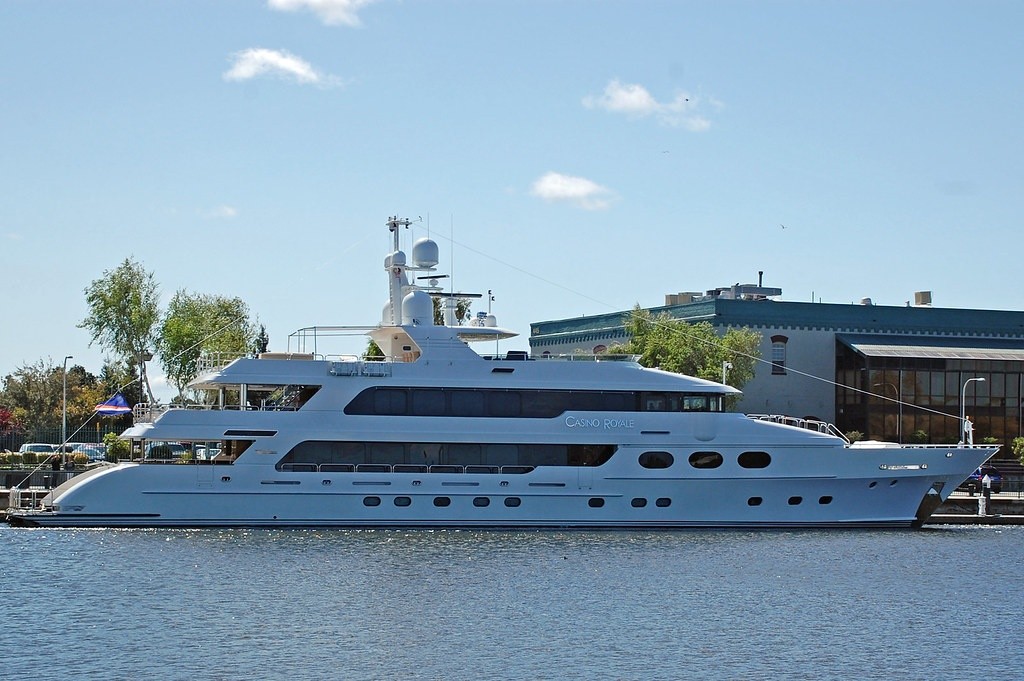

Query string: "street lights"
[[961, 376, 985, 448], [62, 356, 74, 466], [132, 348, 154, 403], [722, 360, 733, 385], [874, 382, 899, 443]]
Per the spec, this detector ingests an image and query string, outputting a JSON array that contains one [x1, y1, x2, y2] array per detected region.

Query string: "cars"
[[18, 439, 221, 463]]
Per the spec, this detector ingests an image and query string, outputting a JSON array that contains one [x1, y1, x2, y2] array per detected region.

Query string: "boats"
[[6, 213, 1002, 531]]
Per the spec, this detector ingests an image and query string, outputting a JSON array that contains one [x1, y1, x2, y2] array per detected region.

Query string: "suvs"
[[954, 464, 1003, 495]]
